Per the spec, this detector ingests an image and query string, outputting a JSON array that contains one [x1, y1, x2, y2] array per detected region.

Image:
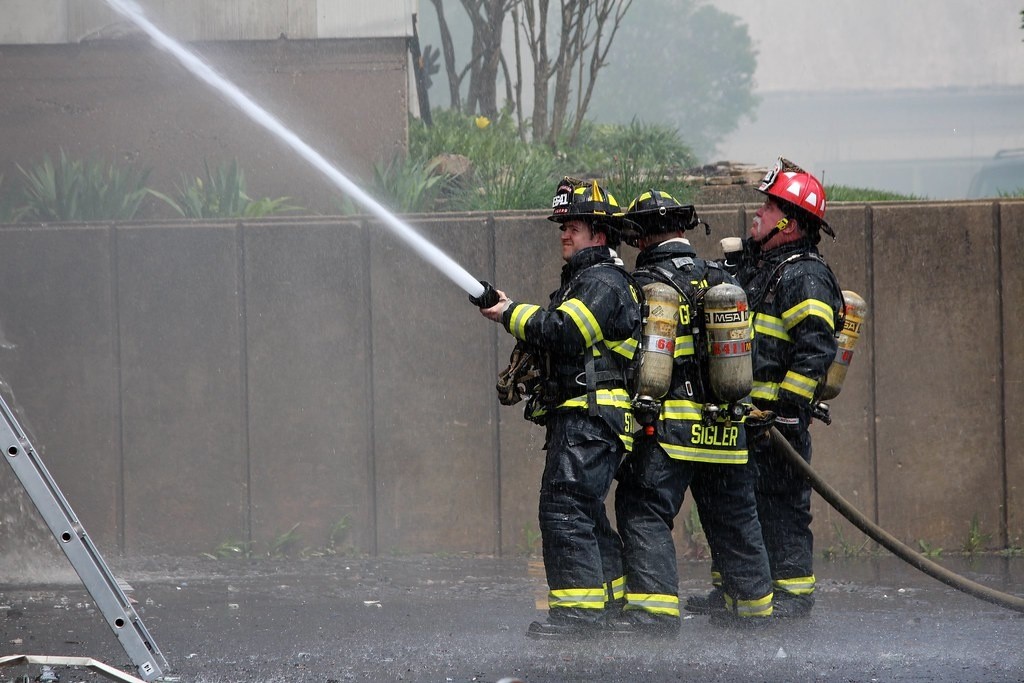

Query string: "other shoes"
[[599, 616, 672, 641], [711, 607, 775, 631], [684, 591, 729, 613], [525, 619, 595, 641], [770, 592, 815, 616]]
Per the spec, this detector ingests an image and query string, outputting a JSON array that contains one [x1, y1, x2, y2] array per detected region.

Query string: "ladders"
[[0, 396, 171, 683]]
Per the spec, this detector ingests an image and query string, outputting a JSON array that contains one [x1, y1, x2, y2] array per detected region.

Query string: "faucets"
[[467, 280, 500, 308]]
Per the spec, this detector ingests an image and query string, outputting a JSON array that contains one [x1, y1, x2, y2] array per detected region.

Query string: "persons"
[[685, 171, 845, 620], [607, 190, 778, 638], [479, 182, 644, 640]]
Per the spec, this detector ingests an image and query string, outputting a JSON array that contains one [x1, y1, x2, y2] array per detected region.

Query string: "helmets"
[[622, 188, 711, 247], [747, 157, 837, 244], [547, 176, 625, 240]]
